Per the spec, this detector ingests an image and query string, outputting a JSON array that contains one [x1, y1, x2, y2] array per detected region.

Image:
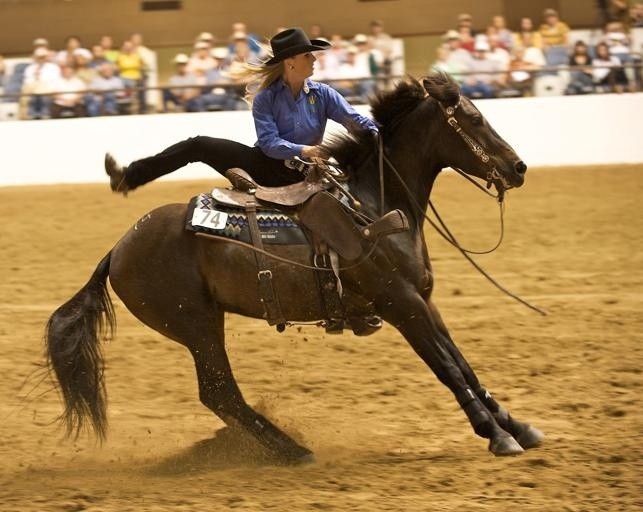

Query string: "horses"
[[20, 68, 546, 464]]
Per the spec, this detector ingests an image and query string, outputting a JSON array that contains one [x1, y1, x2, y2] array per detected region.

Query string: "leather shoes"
[[104, 152, 130, 191]]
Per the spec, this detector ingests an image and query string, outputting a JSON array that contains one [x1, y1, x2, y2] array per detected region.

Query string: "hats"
[[443, 28, 548, 84], [263, 28, 331, 65], [32, 36, 94, 63], [172, 33, 248, 63]]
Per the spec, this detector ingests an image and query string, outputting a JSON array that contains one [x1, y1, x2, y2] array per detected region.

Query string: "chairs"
[[0, 48, 158, 117], [433, 42, 641, 99], [162, 42, 404, 113]]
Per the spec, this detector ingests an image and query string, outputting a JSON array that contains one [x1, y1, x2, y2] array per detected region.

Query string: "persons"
[[104, 28, 380, 197]]
